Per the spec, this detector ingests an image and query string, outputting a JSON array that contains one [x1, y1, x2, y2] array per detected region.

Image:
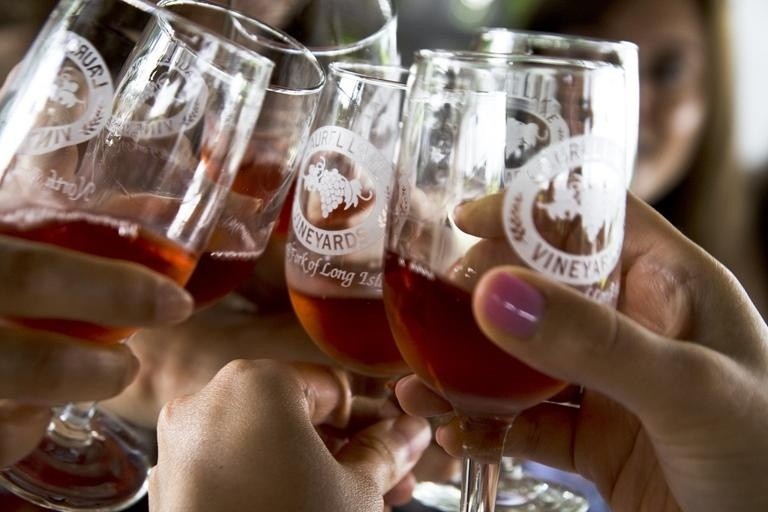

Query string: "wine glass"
[[6, 1, 275, 511], [284, 61, 505, 445], [380, 47, 631, 511], [411, 25, 640, 510], [216, 5, 402, 316], [92, 1, 325, 328]]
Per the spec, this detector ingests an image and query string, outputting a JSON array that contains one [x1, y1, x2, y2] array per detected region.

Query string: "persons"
[[3, 1, 767, 511]]
